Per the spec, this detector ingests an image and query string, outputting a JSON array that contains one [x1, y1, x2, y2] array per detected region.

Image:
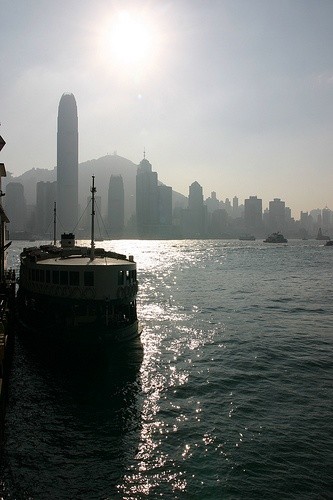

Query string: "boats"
[[18, 173, 143, 345]]
